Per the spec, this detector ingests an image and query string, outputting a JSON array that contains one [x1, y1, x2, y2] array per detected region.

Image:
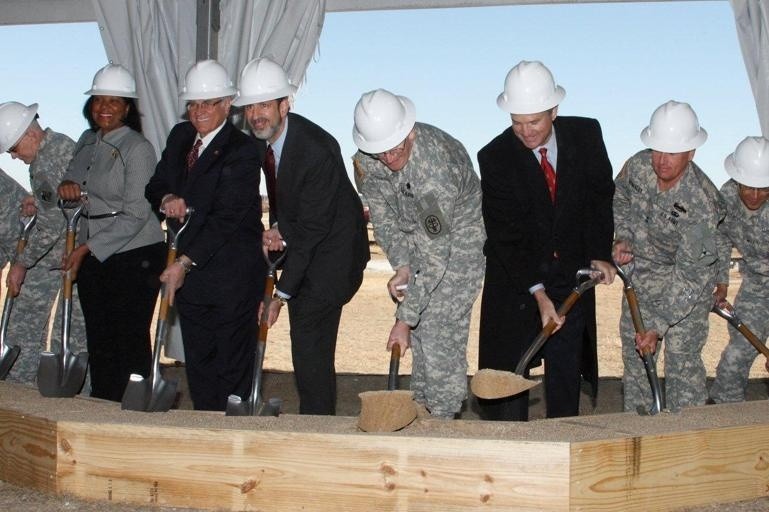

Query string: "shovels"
[[121, 205, 196, 413], [226, 239, 290, 415], [356, 284, 419, 434], [470, 269, 605, 400], [0, 205, 38, 383], [614, 251, 679, 415], [37, 190, 89, 397]]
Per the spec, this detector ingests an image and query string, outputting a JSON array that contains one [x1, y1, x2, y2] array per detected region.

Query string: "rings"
[[168, 208, 177, 216]]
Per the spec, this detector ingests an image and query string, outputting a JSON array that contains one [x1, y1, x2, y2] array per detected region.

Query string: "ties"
[[538, 147, 556, 201], [264, 145, 279, 188], [187, 139, 202, 167]]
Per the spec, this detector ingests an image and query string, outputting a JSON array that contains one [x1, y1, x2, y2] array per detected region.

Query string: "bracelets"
[[175, 256, 193, 274], [273, 291, 287, 307]]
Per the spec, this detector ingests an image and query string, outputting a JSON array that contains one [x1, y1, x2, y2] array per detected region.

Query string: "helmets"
[[724, 136, 769, 189], [177, 58, 238, 102], [0, 100, 39, 155], [352, 89, 418, 155], [84, 63, 141, 100], [640, 98, 708, 154], [230, 56, 298, 109], [496, 59, 566, 116]]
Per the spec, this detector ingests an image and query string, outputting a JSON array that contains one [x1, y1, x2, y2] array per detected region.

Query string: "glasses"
[[5, 134, 27, 153], [185, 102, 225, 111]]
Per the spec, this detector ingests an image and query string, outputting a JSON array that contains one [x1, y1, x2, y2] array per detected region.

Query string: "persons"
[[611, 100, 726, 412], [352, 87, 488, 418], [231, 59, 373, 415], [59, 62, 165, 402], [0, 168, 59, 385], [1, 102, 90, 394], [478, 59, 615, 419], [143, 58, 267, 412], [707, 135, 769, 401]]
